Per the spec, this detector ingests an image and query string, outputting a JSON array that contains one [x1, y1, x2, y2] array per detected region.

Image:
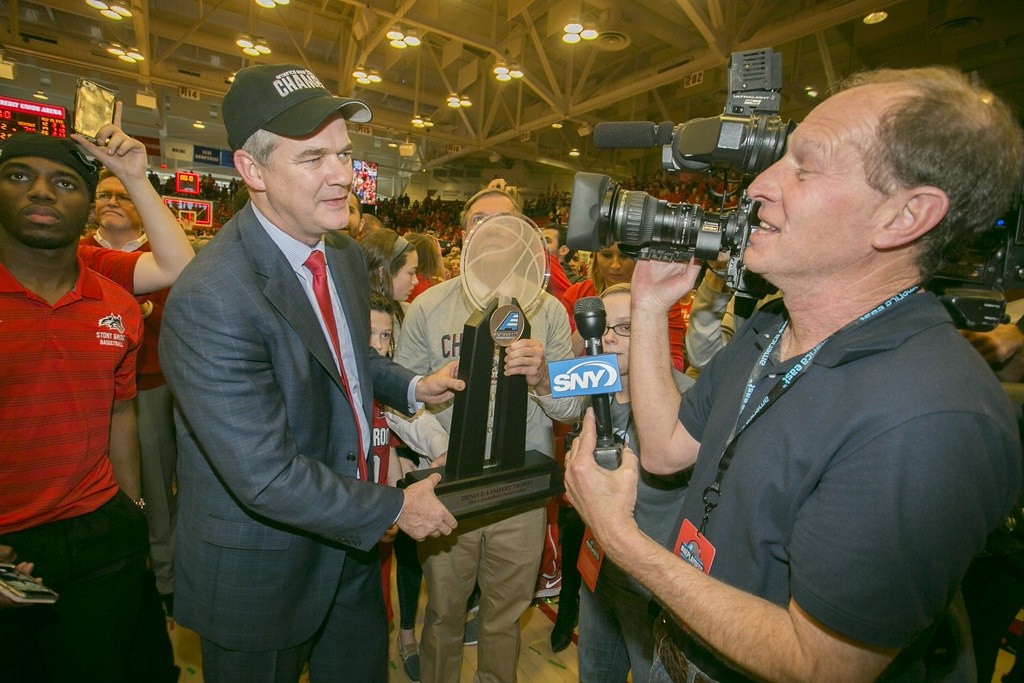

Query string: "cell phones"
[[69, 76, 117, 146], [0, 563, 59, 605]]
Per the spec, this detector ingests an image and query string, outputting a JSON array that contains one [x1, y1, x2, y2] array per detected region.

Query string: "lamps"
[[1, 0, 597, 161]]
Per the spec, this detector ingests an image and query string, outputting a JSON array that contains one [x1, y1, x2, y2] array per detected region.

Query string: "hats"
[[0, 131, 99, 203], [221, 63, 373, 151]]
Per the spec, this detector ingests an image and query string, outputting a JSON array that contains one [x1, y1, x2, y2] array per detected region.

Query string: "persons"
[[578, 281, 697, 683], [385, 182, 580, 683], [340, 179, 448, 683], [959, 314, 1024, 369], [72, 124, 197, 298], [550, 243, 687, 654], [540, 223, 587, 285], [565, 66, 1024, 683], [158, 64, 465, 683], [0, 130, 181, 683], [80, 170, 174, 596]]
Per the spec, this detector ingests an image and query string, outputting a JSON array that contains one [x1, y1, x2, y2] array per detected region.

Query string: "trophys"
[[395, 208, 565, 519]]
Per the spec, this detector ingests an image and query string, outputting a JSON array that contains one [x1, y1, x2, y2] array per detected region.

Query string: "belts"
[[653, 616, 711, 683]]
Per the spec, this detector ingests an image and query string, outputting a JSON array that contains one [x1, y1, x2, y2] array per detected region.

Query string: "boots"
[[551, 506, 585, 653]]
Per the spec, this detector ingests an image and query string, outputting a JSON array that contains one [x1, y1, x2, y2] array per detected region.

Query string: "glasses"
[[601, 324, 631, 337]]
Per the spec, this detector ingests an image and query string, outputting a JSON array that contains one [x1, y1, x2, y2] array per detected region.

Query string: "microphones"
[[547, 297, 624, 466], [593, 119, 677, 149]]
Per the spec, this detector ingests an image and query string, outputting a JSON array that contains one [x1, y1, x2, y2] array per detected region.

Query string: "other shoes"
[[159, 592, 176, 621], [397, 635, 421, 682]]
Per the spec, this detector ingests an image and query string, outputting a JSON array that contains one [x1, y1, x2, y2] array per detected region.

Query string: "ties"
[[301, 250, 368, 483]]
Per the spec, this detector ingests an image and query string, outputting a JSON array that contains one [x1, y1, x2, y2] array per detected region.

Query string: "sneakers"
[[532, 571, 562, 600], [466, 586, 481, 614], [462, 615, 480, 647]]
